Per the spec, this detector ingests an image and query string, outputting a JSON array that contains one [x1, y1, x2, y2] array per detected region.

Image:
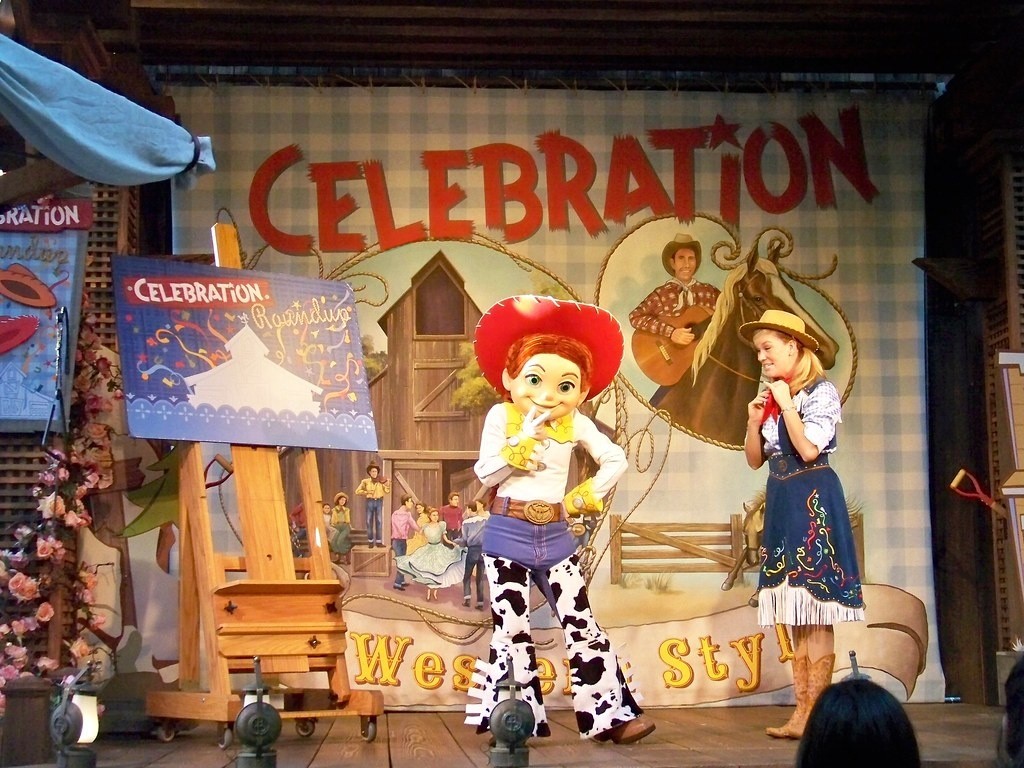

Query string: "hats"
[[739, 309, 820, 352]]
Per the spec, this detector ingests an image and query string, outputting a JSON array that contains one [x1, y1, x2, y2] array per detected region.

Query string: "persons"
[[738, 309, 866, 738], [797, 680, 921, 768], [472, 295, 657, 745], [999, 654, 1024, 768]]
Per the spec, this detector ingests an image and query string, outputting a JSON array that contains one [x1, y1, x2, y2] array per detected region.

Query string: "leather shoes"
[[594, 720, 656, 745]]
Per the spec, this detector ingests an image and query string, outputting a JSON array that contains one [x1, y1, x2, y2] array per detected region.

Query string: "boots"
[[766, 653, 835, 739]]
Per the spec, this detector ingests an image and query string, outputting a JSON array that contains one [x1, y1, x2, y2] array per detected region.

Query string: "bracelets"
[[780, 406, 797, 414]]
